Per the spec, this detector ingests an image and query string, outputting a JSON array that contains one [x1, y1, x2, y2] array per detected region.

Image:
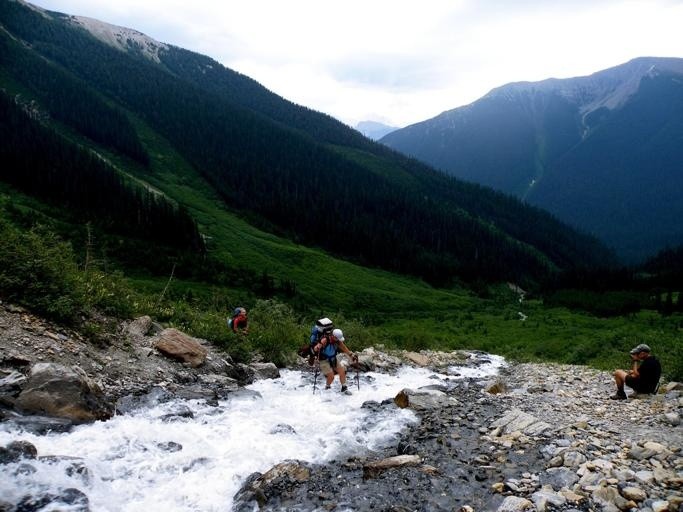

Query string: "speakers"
[[342, 386, 352, 395]]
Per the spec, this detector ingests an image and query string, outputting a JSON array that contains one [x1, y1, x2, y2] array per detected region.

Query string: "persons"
[[233, 308, 249, 335], [609, 343, 662, 400], [313, 328, 358, 396]]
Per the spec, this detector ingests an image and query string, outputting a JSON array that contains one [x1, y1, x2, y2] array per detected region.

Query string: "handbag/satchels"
[[631, 358, 640, 363]]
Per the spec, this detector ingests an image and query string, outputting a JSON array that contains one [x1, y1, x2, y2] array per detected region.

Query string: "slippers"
[[228, 308, 240, 329], [310, 318, 333, 357]]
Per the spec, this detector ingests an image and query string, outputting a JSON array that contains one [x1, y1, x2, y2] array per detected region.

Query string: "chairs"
[[610, 392, 627, 400]]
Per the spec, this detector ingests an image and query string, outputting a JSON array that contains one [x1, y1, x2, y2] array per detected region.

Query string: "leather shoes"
[[350, 354, 357, 362]]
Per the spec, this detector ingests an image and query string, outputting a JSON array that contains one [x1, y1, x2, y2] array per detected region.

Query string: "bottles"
[[632, 344, 650, 353], [333, 329, 345, 342]]
